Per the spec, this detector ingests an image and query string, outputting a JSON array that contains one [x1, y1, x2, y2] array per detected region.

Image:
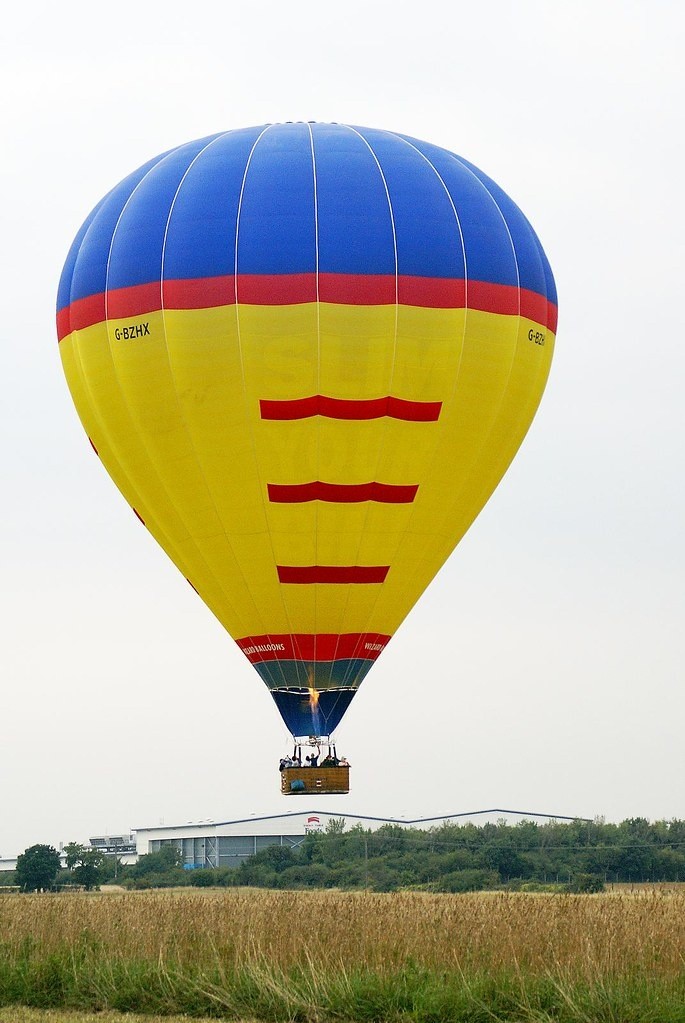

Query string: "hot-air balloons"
[[54, 121, 560, 798]]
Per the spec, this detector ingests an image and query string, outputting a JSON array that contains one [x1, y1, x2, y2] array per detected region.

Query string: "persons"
[[303, 745, 320, 766], [279, 755, 299, 772], [319, 755, 349, 766]]
